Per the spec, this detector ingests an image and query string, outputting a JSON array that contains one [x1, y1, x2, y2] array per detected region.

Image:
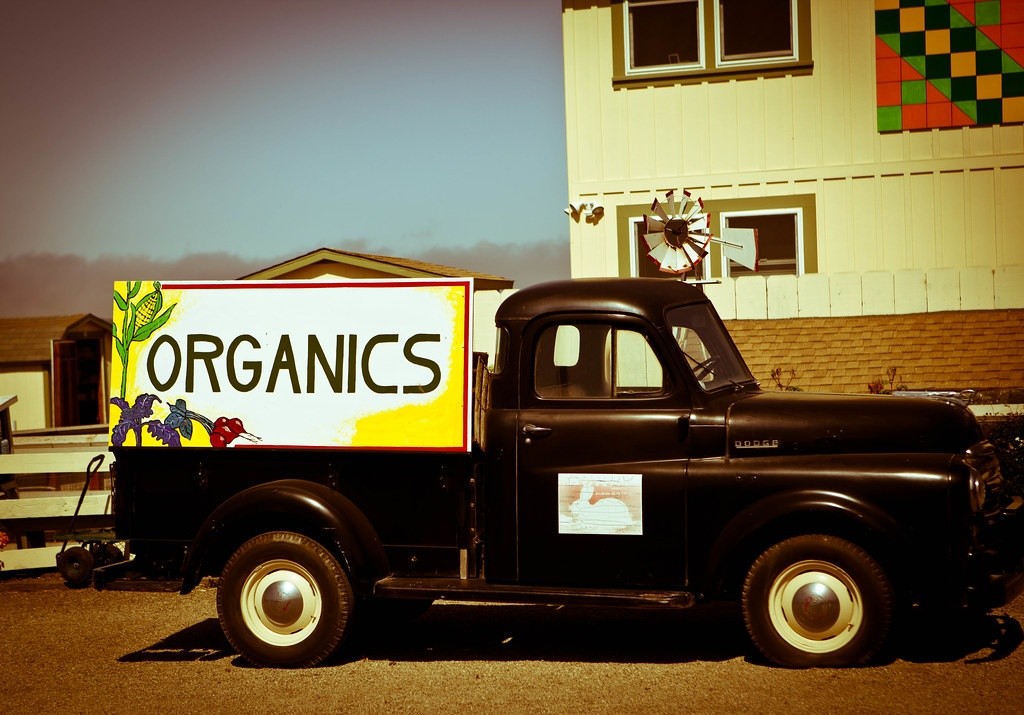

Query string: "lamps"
[[563, 201, 604, 216]]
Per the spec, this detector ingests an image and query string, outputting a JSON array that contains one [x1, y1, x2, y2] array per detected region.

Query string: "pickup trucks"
[[61, 276, 1010, 669]]
